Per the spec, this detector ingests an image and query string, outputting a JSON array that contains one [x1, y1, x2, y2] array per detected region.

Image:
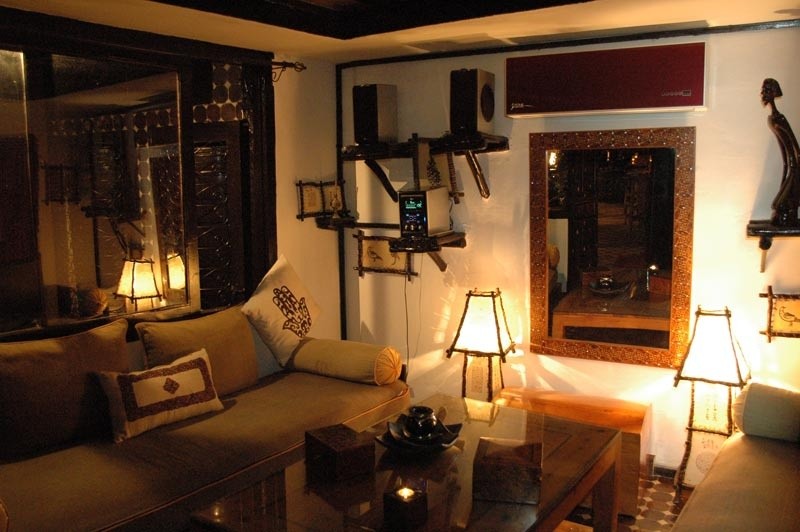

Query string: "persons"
[[759, 76, 800, 228]]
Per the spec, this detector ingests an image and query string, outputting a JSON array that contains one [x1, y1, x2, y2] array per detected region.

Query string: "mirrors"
[[529, 126, 692, 369], [0, 5, 278, 342]]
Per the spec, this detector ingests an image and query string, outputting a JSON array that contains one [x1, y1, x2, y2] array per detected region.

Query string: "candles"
[[395, 488, 415, 499]]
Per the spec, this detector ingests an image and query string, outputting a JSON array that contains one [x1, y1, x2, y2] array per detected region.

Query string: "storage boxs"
[[472, 436, 542, 506], [304, 424, 376, 482]]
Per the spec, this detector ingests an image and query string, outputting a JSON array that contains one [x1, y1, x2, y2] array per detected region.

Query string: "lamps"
[[673, 306, 752, 505], [113, 259, 162, 312], [446, 288, 515, 403], [464, 397, 501, 427], [166, 252, 186, 290]]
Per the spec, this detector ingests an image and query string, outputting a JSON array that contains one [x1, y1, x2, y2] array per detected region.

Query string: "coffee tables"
[[184, 393, 621, 532]]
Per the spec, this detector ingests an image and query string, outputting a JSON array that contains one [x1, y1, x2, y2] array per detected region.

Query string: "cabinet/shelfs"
[[492, 386, 653, 517], [315, 130, 509, 254]]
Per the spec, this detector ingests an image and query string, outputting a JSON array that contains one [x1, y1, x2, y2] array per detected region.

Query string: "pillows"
[[89, 347, 224, 446], [240, 253, 324, 366]]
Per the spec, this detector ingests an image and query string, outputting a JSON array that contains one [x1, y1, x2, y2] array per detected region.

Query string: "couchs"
[[670, 381, 800, 532], [0, 272, 115, 344], [0, 301, 410, 532]]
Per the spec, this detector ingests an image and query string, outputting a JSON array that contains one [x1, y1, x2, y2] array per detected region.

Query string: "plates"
[[376, 413, 463, 457], [588, 280, 632, 298]]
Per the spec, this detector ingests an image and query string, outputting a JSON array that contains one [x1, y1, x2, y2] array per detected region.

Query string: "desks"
[[552, 285, 671, 338]]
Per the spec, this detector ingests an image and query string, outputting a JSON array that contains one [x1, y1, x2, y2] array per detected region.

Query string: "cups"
[[408, 405, 437, 443]]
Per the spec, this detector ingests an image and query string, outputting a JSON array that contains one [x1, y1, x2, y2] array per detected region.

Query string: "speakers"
[[450, 68, 496, 135], [353, 83, 398, 144]]
